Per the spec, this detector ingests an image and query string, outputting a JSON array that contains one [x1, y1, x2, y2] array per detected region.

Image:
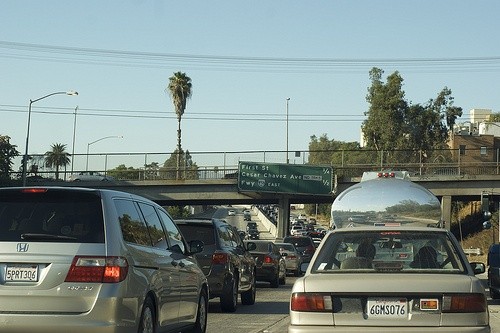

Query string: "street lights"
[[22, 90, 79, 186], [86, 136, 124, 171], [286, 97, 291, 163]]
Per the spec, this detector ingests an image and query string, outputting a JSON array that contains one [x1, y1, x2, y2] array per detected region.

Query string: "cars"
[[243, 239, 288, 288], [273, 243, 305, 276], [287, 228, 492, 333], [227, 208, 261, 241], [486, 241, 500, 299], [256, 204, 328, 248]]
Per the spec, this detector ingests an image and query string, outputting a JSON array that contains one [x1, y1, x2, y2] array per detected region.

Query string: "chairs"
[[339, 257, 373, 271], [409, 246, 441, 269]]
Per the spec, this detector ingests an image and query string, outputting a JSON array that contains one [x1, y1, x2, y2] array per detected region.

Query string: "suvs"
[[281, 234, 317, 263], [0, 181, 210, 333], [173, 217, 258, 313]]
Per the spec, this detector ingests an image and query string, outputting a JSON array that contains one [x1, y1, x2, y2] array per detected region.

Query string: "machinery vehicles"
[[326, 170, 443, 262]]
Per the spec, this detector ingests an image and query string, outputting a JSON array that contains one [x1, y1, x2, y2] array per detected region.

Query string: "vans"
[[347, 215, 367, 224]]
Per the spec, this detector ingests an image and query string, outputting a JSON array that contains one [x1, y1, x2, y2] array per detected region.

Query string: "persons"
[[356, 243, 376, 259], [410, 247, 439, 268]]
